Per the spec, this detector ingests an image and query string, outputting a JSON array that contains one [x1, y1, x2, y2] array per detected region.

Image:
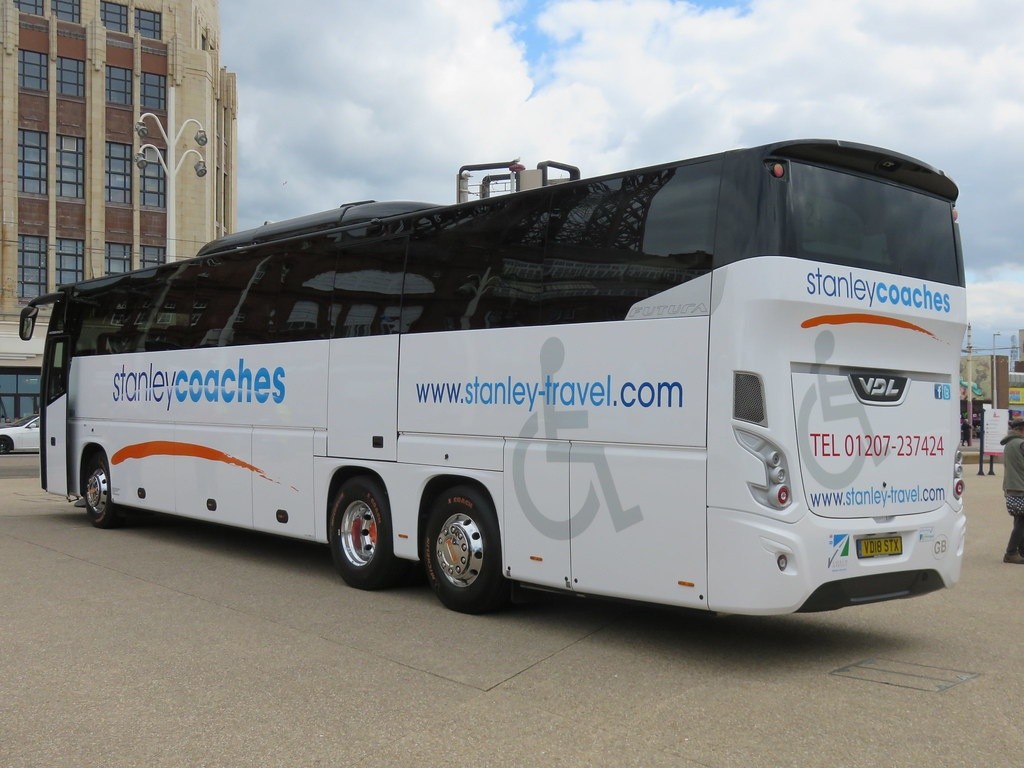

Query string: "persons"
[[961, 419, 972, 446], [999, 417, 1024, 564]]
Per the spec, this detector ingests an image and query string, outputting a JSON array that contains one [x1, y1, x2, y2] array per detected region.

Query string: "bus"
[[18, 136, 968, 620]]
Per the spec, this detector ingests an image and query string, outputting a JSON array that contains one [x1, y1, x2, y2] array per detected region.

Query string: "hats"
[[1011, 416, 1024, 427]]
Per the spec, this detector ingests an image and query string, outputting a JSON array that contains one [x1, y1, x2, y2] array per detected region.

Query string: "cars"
[[0, 414, 40, 455]]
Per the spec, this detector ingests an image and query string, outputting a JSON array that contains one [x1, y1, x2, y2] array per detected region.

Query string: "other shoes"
[[1018, 549, 1024, 557], [1004, 551, 1024, 564]]
